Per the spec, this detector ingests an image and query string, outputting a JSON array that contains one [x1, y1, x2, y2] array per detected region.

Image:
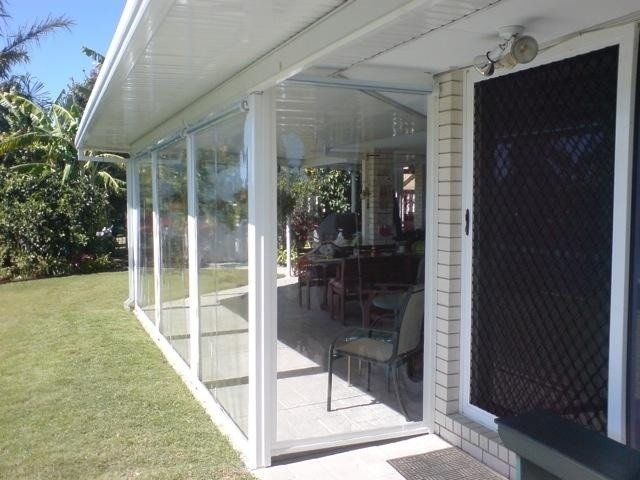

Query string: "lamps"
[[473, 25, 538, 76]]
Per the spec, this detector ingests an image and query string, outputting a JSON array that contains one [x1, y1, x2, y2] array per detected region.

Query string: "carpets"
[[386, 446, 509, 480]]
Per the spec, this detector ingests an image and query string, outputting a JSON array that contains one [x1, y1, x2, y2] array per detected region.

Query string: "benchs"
[[494, 408, 640, 480]]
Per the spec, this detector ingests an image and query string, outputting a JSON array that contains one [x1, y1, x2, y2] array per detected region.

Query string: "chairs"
[[298, 241, 424, 422]]
